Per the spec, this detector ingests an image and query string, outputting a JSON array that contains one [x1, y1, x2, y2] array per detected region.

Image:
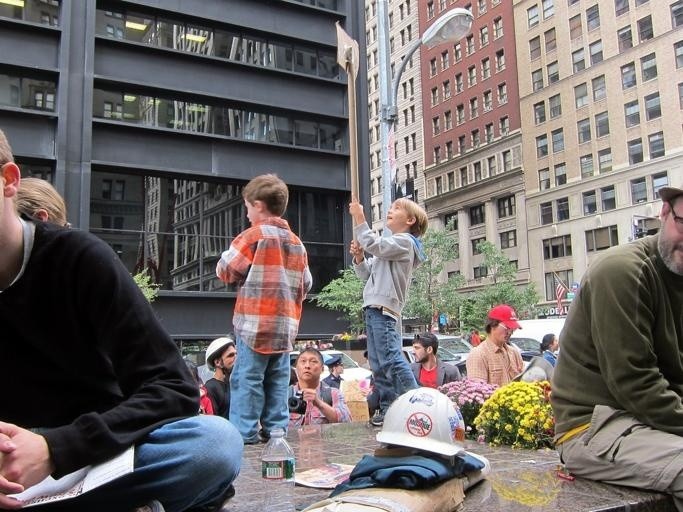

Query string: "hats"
[[287, 335, 545, 380]]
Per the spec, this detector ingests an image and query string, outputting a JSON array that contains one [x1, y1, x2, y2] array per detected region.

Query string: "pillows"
[[374, 386, 466, 456], [205, 336, 234, 371]]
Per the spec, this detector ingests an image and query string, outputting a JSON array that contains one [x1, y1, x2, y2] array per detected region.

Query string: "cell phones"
[[289, 390, 305, 415]]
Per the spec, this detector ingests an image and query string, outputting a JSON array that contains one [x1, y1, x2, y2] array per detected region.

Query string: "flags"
[[550, 274, 568, 317]]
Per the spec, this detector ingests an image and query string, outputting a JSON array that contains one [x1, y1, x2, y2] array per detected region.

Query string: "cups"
[[498, 323, 516, 333], [667, 201, 682, 227], [413, 333, 429, 340]]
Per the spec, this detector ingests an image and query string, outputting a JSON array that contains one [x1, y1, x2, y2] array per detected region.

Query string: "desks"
[[374, 2, 474, 227]]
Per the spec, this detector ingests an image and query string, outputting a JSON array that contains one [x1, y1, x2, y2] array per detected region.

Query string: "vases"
[[437, 377, 555, 450]]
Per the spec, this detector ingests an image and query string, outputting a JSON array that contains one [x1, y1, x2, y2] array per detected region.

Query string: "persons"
[[539, 333, 559, 367], [204, 337, 237, 421], [184, 359, 214, 415], [364, 351, 380, 418], [0, 128, 246, 511], [321, 356, 344, 389], [344, 191, 434, 429], [16, 174, 73, 229], [215, 170, 314, 446], [466, 305, 525, 388], [465, 326, 482, 347], [284, 346, 352, 426], [409, 332, 462, 389], [545, 177, 683, 509]]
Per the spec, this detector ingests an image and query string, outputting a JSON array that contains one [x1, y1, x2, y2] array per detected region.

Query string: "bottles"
[[659, 167, 683, 202], [325, 357, 344, 367], [488, 305, 522, 330]]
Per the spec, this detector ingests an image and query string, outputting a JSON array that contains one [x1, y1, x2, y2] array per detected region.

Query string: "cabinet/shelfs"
[[258, 426, 298, 512]]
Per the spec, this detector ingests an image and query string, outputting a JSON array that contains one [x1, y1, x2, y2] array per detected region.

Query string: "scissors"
[[334, 19, 362, 254]]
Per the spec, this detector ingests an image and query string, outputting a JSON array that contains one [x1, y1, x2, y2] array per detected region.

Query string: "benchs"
[[550, 274, 568, 317]]
